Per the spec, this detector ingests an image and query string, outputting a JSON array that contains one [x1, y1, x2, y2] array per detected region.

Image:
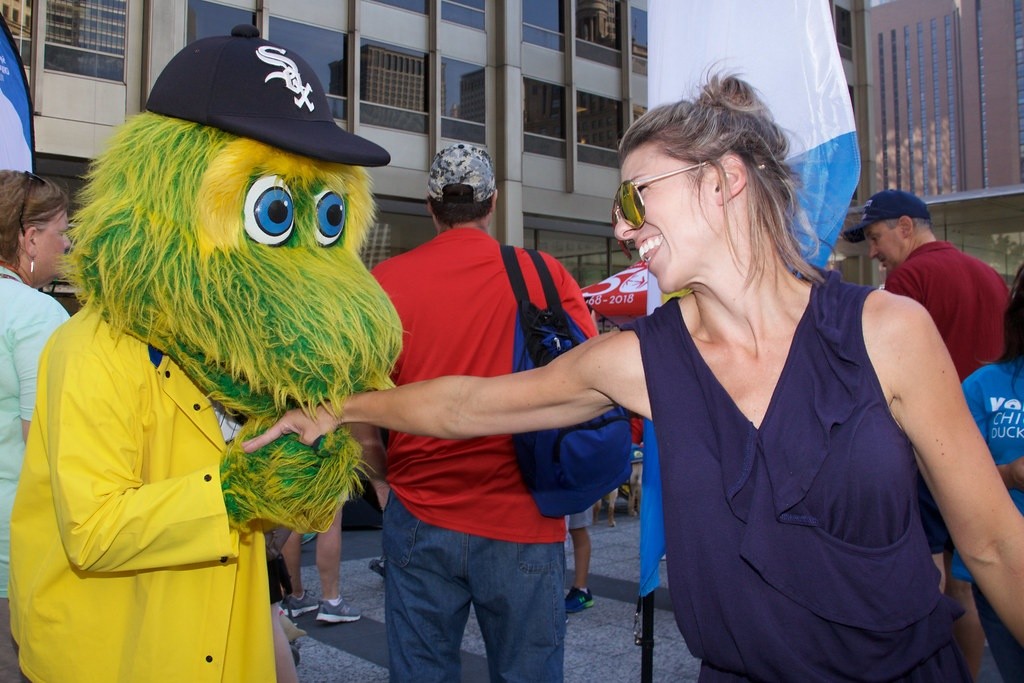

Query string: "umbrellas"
[[580, 260, 648, 319]]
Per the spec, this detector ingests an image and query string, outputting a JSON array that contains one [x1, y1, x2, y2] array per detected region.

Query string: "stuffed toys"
[[8, 23, 402, 683]]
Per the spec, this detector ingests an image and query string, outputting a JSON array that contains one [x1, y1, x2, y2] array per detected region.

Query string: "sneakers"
[[565, 586, 595, 613], [283, 592, 319, 618], [316, 598, 361, 623]]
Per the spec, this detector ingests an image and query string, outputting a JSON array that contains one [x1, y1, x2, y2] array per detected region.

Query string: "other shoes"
[[300, 533, 318, 545], [565, 613, 569, 623]]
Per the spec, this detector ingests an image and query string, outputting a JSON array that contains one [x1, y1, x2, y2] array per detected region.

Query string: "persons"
[[264, 506, 362, 683], [842, 189, 1024, 683], [371, 143, 600, 683], [241, 76, 1024, 683], [565, 505, 595, 624], [0, 170, 72, 683]]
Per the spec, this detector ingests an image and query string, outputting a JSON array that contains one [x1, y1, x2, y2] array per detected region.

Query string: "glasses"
[[612, 159, 765, 231], [19, 170, 46, 237]]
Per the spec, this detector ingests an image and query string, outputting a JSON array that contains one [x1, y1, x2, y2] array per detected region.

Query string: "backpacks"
[[499, 245, 635, 518]]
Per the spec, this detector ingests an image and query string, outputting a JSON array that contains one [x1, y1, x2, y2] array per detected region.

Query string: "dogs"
[[593, 441, 643, 526]]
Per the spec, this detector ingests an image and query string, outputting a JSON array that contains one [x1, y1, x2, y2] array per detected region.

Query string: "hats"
[[841, 189, 931, 244], [425, 142, 496, 205]]
